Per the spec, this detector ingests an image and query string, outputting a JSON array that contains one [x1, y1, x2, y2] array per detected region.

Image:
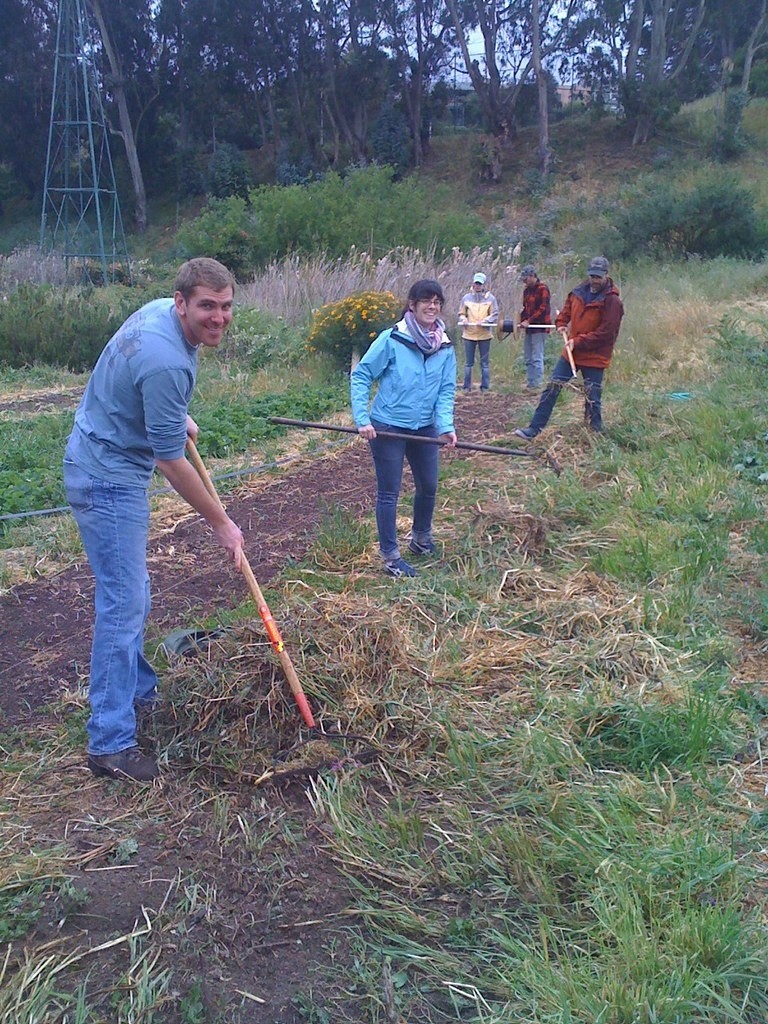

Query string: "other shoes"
[[515, 427, 542, 439], [87, 747, 161, 780]]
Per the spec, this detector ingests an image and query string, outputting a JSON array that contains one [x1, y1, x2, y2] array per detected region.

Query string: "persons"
[[517, 262, 551, 396], [514, 257, 624, 449], [63, 257, 245, 784], [351, 279, 457, 577], [457, 272, 499, 392]]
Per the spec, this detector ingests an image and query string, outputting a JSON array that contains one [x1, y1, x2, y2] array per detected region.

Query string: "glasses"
[[415, 298, 442, 307]]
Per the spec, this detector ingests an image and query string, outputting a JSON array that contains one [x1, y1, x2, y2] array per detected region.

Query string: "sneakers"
[[384, 559, 418, 579], [406, 532, 439, 557]]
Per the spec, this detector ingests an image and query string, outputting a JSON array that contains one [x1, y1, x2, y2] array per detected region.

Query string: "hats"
[[473, 273, 487, 285], [587, 256, 609, 279]]
